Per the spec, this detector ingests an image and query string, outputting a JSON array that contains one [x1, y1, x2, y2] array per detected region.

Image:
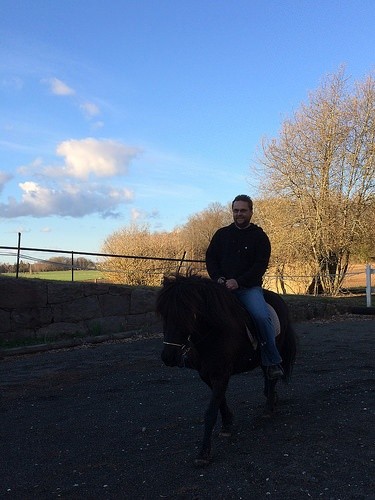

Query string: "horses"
[[155, 273, 297, 467]]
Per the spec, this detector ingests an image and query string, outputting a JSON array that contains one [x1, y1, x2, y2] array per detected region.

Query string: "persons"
[[205, 195, 285, 378]]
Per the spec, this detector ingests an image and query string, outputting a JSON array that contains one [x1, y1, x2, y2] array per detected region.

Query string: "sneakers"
[[268, 365, 284, 378]]
